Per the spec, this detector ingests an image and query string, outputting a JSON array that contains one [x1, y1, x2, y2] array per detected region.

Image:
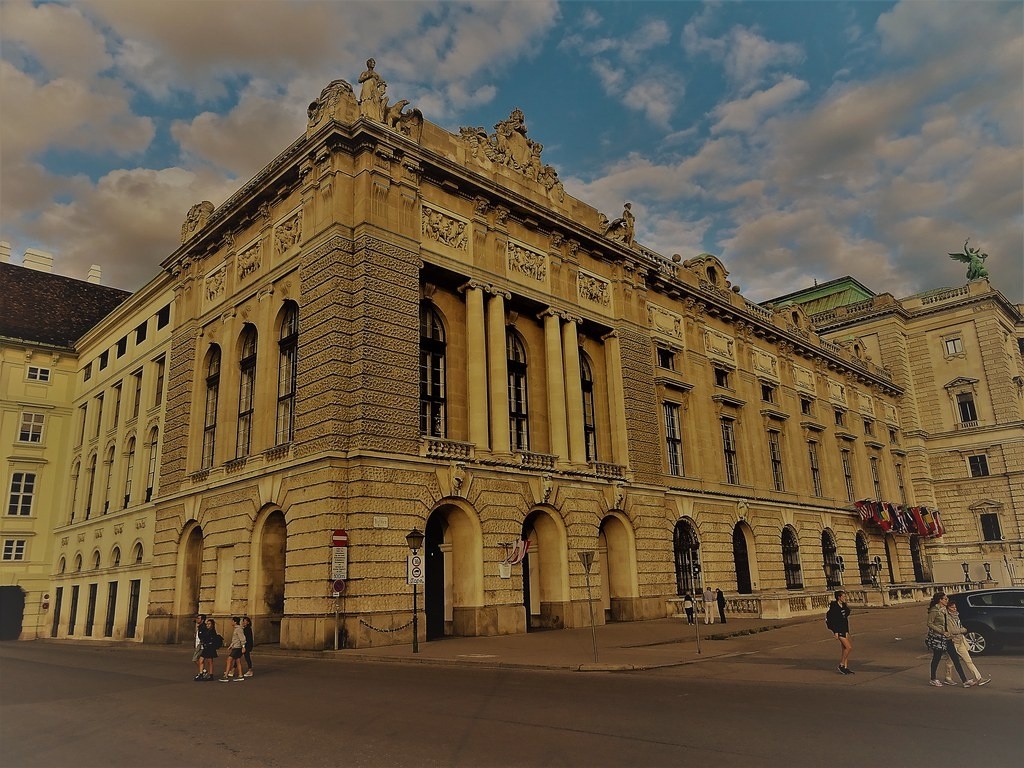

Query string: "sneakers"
[[944, 678, 958, 686], [846, 668, 856, 675], [962, 681, 977, 688], [838, 665, 847, 674], [978, 678, 991, 685], [929, 679, 943, 686]]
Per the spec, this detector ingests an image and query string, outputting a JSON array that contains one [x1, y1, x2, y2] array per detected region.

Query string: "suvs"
[[928, 588, 1024, 656]]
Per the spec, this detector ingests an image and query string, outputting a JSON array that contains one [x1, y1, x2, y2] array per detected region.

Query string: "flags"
[[853, 496, 948, 539], [503, 537, 534, 569]]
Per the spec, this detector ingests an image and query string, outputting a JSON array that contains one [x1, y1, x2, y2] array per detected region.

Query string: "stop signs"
[[331, 529, 349, 547]]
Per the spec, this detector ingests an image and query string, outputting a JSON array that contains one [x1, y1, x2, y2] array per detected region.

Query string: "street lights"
[[404, 526, 424, 653]]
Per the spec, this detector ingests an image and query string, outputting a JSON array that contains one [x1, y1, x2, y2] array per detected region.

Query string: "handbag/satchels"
[[925, 631, 946, 651], [963, 636, 971, 651]]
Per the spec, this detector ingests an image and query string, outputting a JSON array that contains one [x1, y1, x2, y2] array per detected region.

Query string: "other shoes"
[[193, 673, 204, 681], [203, 669, 207, 676], [218, 677, 229, 682], [233, 676, 245, 682], [704, 621, 708, 624], [243, 671, 254, 677], [711, 621, 714, 624], [224, 671, 234, 676], [204, 674, 214, 682]]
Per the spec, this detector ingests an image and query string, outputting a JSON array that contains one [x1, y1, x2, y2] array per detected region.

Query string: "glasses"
[[941, 598, 948, 601]]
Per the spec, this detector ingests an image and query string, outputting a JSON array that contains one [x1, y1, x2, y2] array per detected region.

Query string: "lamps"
[[868, 561, 880, 588], [821, 561, 835, 591], [983, 561, 993, 580], [961, 561, 971, 582]]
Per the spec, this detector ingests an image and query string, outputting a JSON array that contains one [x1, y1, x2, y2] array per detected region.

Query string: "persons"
[[714, 587, 728, 624], [420, 206, 614, 308], [682, 591, 697, 627], [618, 198, 637, 248], [218, 616, 254, 687], [357, 57, 388, 123], [824, 590, 857, 676], [700, 586, 716, 624], [191, 613, 217, 682], [923, 593, 992, 688], [948, 238, 991, 284], [204, 210, 303, 303]]
